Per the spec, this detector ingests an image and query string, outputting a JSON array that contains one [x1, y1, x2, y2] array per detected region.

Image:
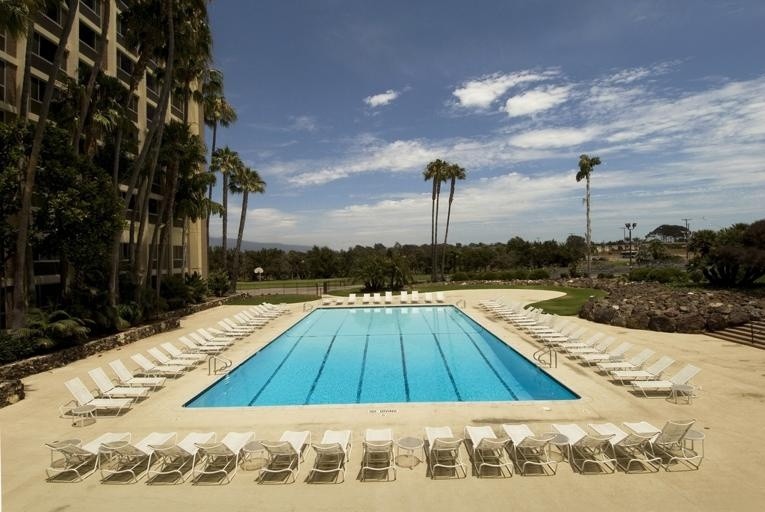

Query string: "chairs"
[[310, 430, 351, 484], [52, 298, 293, 429], [317, 288, 449, 306], [552, 418, 702, 474], [361, 428, 397, 482], [474, 290, 705, 406], [44, 431, 256, 487], [259, 431, 311, 482], [423, 426, 467, 481], [464, 423, 558, 478]]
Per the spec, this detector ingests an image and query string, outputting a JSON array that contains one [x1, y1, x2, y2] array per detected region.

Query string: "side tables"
[[242, 441, 265, 471], [394, 437, 424, 470], [542, 432, 569, 463], [683, 429, 705, 458]]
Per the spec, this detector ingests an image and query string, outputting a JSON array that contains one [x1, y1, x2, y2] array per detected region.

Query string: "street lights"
[[625, 222, 638, 282]]
